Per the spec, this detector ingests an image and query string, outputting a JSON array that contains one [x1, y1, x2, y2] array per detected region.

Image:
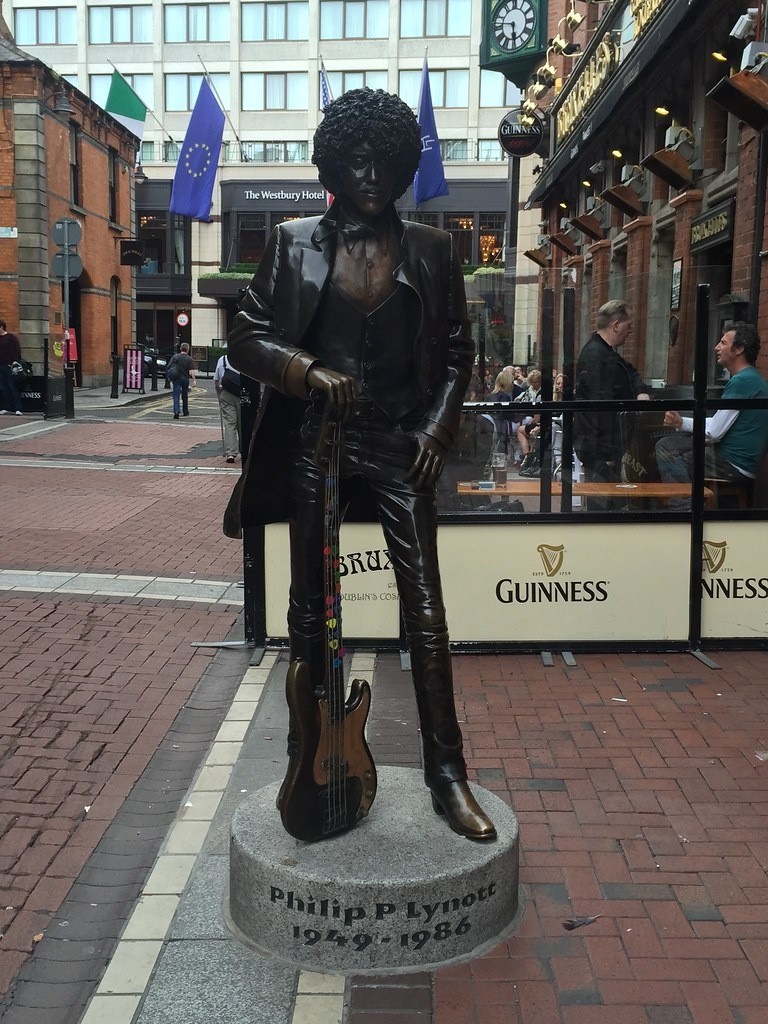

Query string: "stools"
[[706, 481, 748, 510]]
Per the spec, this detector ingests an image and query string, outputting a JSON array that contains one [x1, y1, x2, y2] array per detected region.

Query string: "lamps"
[[39, 83, 74, 123], [93, 116, 109, 134], [516, 0, 768, 267], [133, 161, 148, 185]]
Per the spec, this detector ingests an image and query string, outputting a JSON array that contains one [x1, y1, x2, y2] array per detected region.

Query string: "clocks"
[[488, 0, 537, 54]]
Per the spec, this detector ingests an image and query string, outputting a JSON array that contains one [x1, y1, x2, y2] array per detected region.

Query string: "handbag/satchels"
[[167, 363, 180, 381], [222, 368, 241, 397]]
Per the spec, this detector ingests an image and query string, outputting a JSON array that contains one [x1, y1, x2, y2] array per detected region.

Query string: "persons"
[[654, 325, 768, 513], [213, 352, 243, 463], [458, 359, 569, 511], [165, 343, 198, 420], [573, 300, 651, 510], [0, 319, 25, 416], [224, 81, 498, 844]]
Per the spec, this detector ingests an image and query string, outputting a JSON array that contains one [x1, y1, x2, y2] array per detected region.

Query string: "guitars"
[[273, 384, 381, 844]]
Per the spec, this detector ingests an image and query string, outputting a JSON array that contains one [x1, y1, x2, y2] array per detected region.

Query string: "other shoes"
[[226, 456, 235, 464], [173, 412, 179, 419], [183, 411, 190, 416], [15, 410, 22, 415], [0, 409, 13, 415]]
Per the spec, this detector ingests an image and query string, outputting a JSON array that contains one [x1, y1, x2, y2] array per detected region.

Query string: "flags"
[[105, 69, 148, 169], [405, 61, 452, 210], [318, 71, 337, 208], [170, 75, 226, 222]]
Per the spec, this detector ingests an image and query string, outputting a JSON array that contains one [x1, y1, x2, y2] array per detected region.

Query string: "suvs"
[[132, 342, 168, 378]]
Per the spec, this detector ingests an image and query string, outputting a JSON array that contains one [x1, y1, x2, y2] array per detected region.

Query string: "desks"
[[450, 479, 714, 513]]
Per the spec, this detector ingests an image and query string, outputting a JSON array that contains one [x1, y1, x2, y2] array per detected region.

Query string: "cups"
[[471, 480, 480, 490], [651, 379, 665, 388], [492, 453, 507, 486]]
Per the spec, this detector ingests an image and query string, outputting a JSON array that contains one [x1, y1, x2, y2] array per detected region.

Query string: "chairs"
[[522, 414, 574, 481]]
